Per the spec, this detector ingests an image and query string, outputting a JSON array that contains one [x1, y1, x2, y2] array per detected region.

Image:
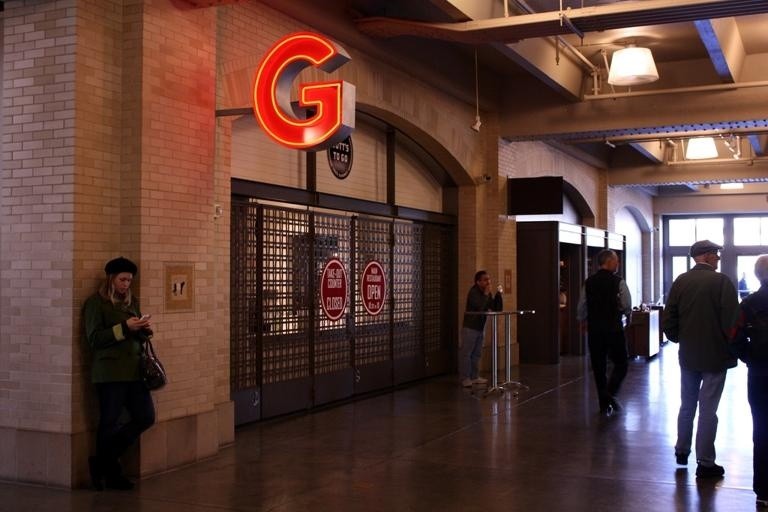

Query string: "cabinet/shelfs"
[[632, 309, 660, 360], [605, 230, 627, 284], [652, 304, 668, 345], [517, 221, 583, 366], [582, 224, 607, 281]]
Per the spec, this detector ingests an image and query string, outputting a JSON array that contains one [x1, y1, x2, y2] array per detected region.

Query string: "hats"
[[104, 256, 137, 276], [690, 240, 723, 257]]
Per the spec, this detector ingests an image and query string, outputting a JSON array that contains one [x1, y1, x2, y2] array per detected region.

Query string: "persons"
[[738, 256, 768, 510], [738, 272, 750, 298], [80, 256, 166, 493], [577, 249, 631, 418], [663, 240, 738, 476], [457, 270, 504, 387]]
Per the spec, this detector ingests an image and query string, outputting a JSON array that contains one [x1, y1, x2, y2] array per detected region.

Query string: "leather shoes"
[[602, 392, 623, 410], [600, 406, 612, 415]]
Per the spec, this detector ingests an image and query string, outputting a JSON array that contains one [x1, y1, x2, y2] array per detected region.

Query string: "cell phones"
[[497, 285, 503, 295], [139, 314, 152, 323]]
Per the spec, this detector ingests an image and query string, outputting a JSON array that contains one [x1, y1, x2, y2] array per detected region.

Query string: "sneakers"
[[462, 377, 472, 387], [696, 463, 724, 477], [472, 378, 488, 384], [756, 496, 768, 506], [676, 454, 688, 465]]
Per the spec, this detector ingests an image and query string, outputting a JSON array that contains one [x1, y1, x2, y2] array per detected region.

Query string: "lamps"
[[686, 137, 718, 160], [720, 183, 744, 190], [607, 47, 660, 86]]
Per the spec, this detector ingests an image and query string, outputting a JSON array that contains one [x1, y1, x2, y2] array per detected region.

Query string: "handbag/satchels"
[[142, 338, 167, 391]]
